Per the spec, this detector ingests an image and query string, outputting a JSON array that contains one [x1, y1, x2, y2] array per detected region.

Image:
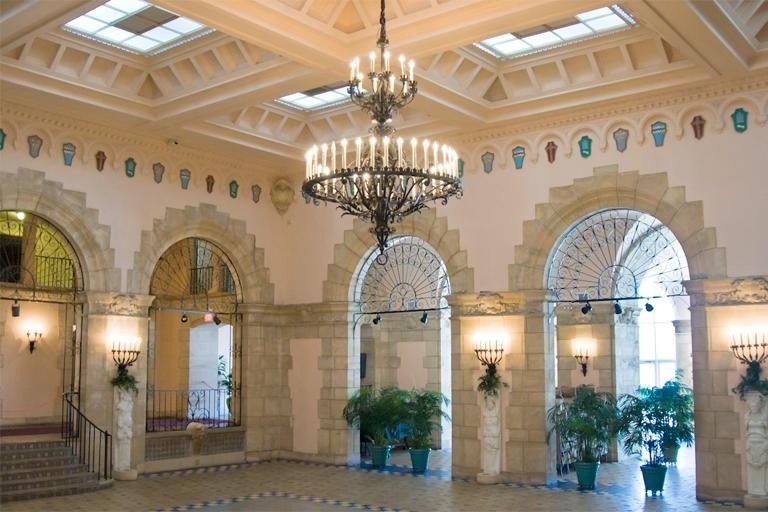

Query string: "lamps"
[[300, 0, 466, 269], [26, 330, 42, 354], [573, 338, 599, 376], [181, 313, 224, 327], [363, 309, 448, 324], [580, 300, 659, 316], [10, 301, 21, 319], [728, 329, 768, 383], [473, 339, 503, 376], [107, 337, 141, 387]]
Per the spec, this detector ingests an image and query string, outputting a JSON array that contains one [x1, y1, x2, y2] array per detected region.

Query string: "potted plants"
[[542, 378, 694, 495], [342, 384, 452, 472], [216, 355, 233, 413]]
